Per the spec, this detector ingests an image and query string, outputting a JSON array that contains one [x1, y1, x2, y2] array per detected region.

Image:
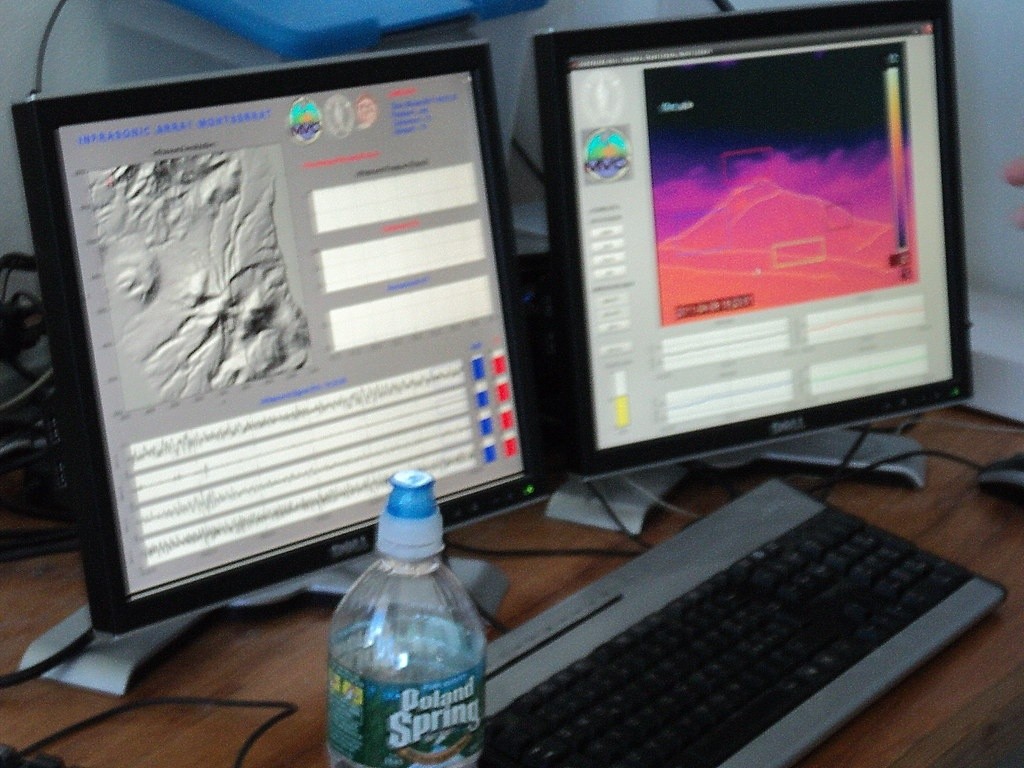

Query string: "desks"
[[0, 399, 1024, 768]]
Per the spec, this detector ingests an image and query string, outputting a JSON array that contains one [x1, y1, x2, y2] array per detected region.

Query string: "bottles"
[[325, 471, 486, 768]]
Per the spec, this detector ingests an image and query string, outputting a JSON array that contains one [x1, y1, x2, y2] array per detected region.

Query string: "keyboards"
[[477, 478, 1008, 768]]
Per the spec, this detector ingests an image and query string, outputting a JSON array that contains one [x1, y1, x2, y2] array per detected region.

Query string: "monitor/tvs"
[[530, 0, 976, 538], [13, 38, 551, 698]]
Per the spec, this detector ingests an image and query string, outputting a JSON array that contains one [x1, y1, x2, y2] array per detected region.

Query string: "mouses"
[[978, 454, 1024, 507]]
